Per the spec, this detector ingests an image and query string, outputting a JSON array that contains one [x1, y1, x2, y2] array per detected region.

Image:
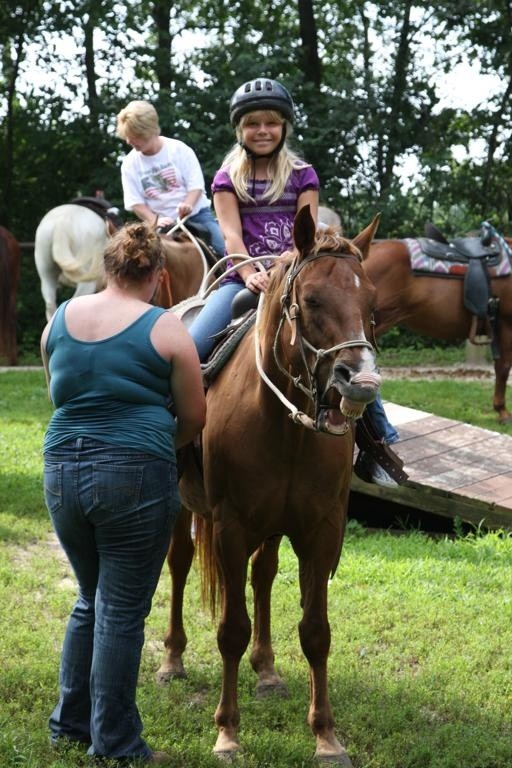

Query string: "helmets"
[[227, 77, 294, 128]]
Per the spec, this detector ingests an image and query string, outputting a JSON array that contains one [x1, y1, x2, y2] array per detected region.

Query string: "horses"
[[359, 236, 512, 427], [32, 202, 120, 322], [104, 212, 226, 311], [165, 203, 385, 768], [0, 225, 21, 366]]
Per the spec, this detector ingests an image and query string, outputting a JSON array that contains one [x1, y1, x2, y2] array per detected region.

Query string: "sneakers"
[[143, 750, 173, 767], [361, 451, 400, 490]]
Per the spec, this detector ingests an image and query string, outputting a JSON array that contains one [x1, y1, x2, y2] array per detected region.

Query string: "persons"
[[30, 220, 211, 767], [182, 77, 400, 490], [115, 100, 234, 280]]
